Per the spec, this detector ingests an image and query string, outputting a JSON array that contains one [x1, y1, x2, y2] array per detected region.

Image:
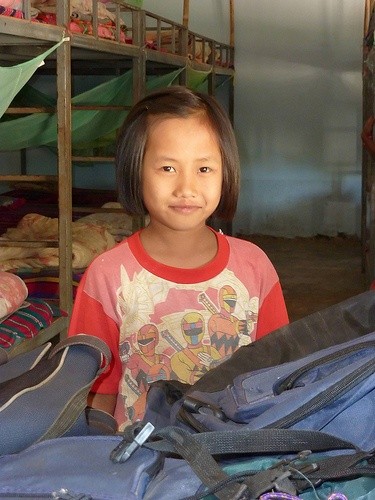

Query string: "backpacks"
[[109, 288, 375, 500]]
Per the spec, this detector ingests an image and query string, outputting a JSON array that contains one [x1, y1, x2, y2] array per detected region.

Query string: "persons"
[[66, 87, 291, 436]]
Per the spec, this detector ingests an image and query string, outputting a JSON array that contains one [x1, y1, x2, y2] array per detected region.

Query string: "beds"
[[0, 0, 234, 367]]
